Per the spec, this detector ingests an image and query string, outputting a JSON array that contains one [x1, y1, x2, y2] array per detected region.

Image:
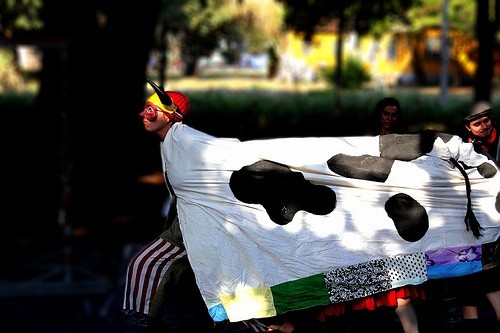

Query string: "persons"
[[114, 77, 500, 333], [362, 97, 403, 137]]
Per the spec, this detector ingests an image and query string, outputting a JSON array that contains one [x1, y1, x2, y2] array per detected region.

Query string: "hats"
[[463, 101, 493, 122], [144, 77, 191, 122]]
